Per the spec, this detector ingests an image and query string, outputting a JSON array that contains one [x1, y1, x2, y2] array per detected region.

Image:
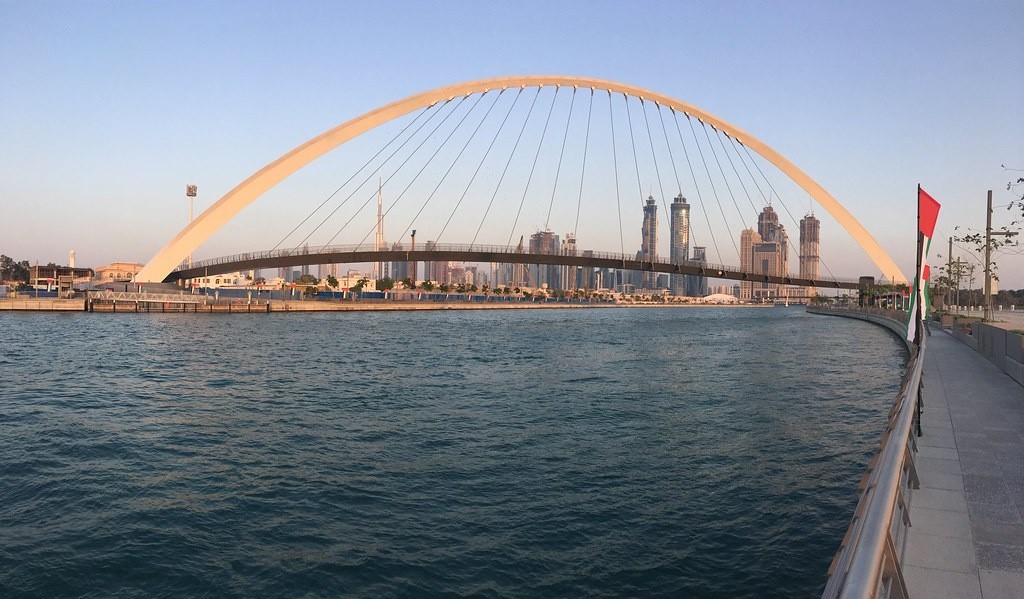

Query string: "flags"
[[878, 284, 914, 310], [906, 186, 942, 345]]
